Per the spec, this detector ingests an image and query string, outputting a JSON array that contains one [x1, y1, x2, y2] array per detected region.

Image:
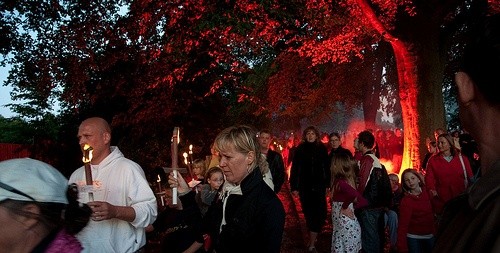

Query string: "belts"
[[408, 234, 434, 239]]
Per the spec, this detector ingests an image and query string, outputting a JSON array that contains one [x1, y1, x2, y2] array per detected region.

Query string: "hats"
[[0, 158, 71, 205]]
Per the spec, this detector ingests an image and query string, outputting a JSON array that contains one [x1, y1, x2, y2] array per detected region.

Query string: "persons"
[[68, 117, 158, 253], [329, 127, 402, 253], [329, 150, 369, 253], [0, 157, 92, 253], [285, 126, 332, 253], [397, 168, 437, 253], [420, 126, 482, 218], [434, 35, 500, 253], [168, 125, 286, 253]]
